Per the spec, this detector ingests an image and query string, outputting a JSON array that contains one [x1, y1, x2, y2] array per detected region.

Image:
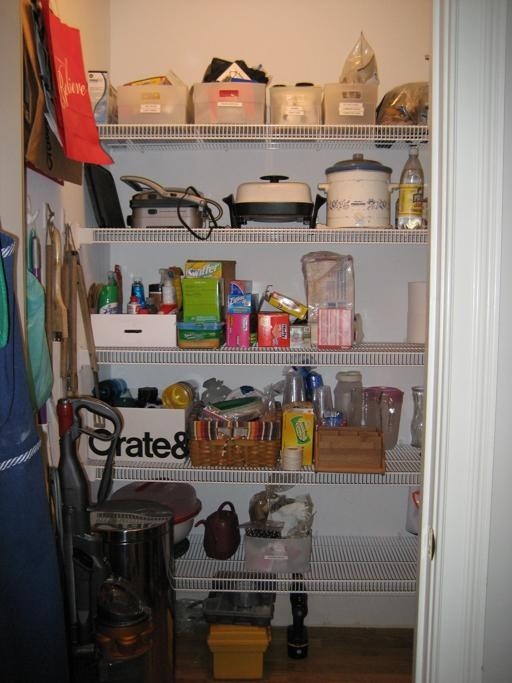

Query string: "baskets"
[[186, 400, 281, 468]]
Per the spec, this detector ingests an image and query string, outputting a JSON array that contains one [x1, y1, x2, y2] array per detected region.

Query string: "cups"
[[281, 373, 333, 421], [161, 382, 195, 408]]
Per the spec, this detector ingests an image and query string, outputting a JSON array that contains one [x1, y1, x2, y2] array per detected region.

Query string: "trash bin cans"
[[90, 499, 176, 683]]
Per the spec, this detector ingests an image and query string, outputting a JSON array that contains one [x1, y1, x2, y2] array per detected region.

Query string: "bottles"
[[99, 264, 162, 314], [398, 148, 424, 228], [334, 371, 363, 426], [410, 386, 426, 448]]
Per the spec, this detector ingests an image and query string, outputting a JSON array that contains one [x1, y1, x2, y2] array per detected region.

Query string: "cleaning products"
[[131, 275, 145, 308], [157, 268, 177, 315]]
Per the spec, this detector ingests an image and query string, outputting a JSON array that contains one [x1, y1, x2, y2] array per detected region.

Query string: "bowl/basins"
[[110, 482, 201, 545]]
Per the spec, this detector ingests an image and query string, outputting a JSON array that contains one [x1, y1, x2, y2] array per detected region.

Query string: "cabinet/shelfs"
[[65, 119, 431, 597]]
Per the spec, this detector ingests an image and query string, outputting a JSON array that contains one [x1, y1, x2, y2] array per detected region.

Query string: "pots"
[[222, 152, 398, 229]]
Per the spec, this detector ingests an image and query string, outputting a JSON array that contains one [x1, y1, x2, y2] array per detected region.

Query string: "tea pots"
[[365, 385, 404, 450], [195, 502, 241, 559]]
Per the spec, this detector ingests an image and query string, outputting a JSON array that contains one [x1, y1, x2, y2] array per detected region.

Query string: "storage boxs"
[[115, 79, 378, 126], [85, 405, 192, 464]]
